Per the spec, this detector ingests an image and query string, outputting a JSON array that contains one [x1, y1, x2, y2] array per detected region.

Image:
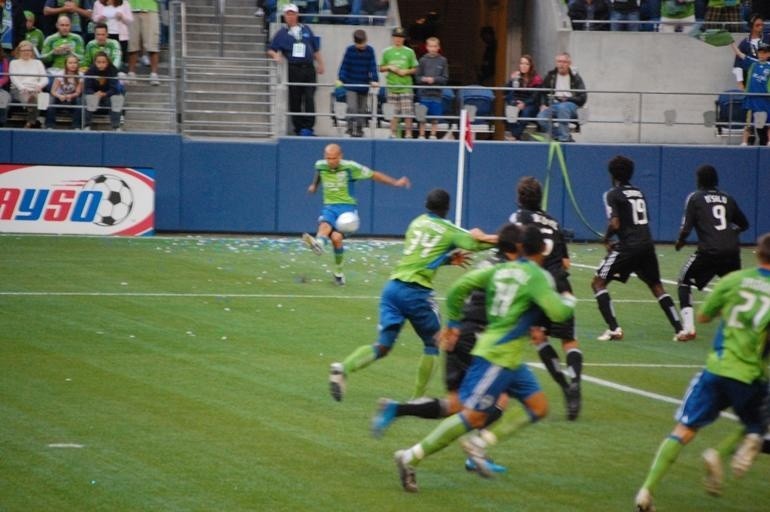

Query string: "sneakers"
[[302, 233, 323, 256], [113, 127, 122, 132], [128, 72, 138, 86], [300, 128, 314, 135], [84, 124, 91, 129], [37, 119, 48, 130], [390, 136, 437, 141], [26, 121, 41, 128], [333, 274, 345, 285], [597, 327, 625, 340], [674, 330, 696, 341], [149, 73, 159, 86], [633, 434, 764, 510], [324, 358, 583, 494]]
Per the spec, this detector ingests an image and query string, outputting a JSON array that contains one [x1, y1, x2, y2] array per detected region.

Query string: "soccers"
[[337, 212, 359, 234]]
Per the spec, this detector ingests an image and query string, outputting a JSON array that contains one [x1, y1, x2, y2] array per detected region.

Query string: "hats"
[[284, 4, 299, 12], [391, 26, 406, 35]]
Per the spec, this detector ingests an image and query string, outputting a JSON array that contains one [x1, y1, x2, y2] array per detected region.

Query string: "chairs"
[[0, 0, 174, 126], [710, 89, 749, 144], [329, 87, 579, 140]]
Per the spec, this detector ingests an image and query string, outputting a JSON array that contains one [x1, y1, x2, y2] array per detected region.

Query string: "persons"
[[500, 176, 586, 422], [634, 231, 769, 510], [672, 164, 750, 343], [414, 36, 450, 141], [565, 1, 770, 34], [0, 0, 165, 134], [731, 13, 770, 93], [376, 26, 421, 141], [338, 28, 379, 137], [499, 54, 541, 140], [389, 225, 580, 493], [255, 1, 498, 86], [701, 423, 750, 494], [738, 42, 770, 147], [536, 51, 588, 142], [299, 143, 412, 286], [325, 187, 499, 404], [266, 4, 326, 139], [368, 222, 523, 473], [588, 153, 691, 345]]
